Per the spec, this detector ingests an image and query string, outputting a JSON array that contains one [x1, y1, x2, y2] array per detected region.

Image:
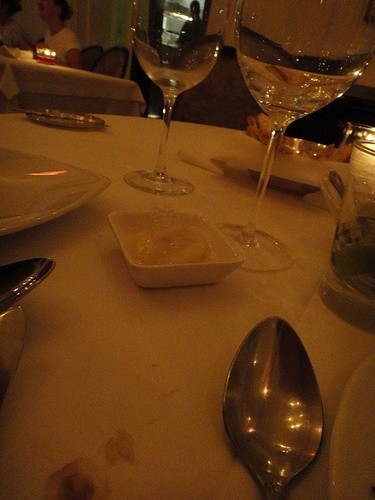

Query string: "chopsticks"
[[9, 104, 112, 128]]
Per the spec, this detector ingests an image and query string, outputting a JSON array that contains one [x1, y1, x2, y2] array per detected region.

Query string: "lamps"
[[36, 48, 56, 65]]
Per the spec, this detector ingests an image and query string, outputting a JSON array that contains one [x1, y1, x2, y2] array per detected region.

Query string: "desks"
[[0, 112, 375, 500], [0, 49, 146, 118]]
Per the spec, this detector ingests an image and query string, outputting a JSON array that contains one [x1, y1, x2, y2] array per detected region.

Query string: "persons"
[[0, 0, 83, 69], [171, 0, 205, 60]]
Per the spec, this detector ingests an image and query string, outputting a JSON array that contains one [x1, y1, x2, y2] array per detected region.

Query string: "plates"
[[328, 347, 375, 500], [248, 167, 323, 200], [25, 108, 105, 131], [0, 144, 113, 238]]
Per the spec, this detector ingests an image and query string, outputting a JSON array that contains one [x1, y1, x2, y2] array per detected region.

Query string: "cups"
[[318, 139, 375, 338], [337, 121, 375, 149]]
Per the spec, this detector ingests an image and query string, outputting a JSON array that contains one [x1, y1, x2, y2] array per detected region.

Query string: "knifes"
[[0, 304, 27, 409]]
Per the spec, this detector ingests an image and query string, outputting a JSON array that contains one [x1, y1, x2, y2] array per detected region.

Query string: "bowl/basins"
[[107, 208, 245, 289]]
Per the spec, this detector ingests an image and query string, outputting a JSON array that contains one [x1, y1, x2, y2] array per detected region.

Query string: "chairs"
[[92, 46, 129, 79], [81, 44, 103, 71]]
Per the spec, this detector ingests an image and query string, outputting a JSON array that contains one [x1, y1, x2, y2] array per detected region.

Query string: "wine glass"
[[232, 0, 375, 273], [127, 0, 230, 198]]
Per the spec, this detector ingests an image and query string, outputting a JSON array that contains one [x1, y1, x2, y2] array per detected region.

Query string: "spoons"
[[222, 314, 325, 500], [0, 257, 57, 317]]
